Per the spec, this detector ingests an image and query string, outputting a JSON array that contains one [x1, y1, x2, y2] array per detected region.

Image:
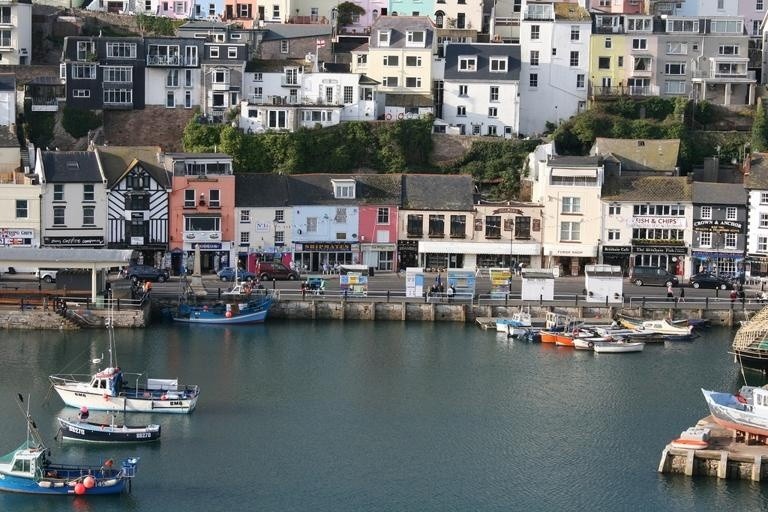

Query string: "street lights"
[[596, 237, 602, 265], [272, 218, 280, 262], [716, 239, 721, 276], [508, 218, 515, 295]]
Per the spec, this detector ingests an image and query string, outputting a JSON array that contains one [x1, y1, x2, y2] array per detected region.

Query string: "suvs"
[[124, 265, 169, 284]]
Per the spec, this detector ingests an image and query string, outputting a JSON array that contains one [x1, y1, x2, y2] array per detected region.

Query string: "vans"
[[257, 262, 299, 281], [629, 265, 678, 287]]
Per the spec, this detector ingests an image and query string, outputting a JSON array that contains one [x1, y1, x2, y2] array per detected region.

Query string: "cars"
[[36, 268, 68, 282], [689, 271, 734, 290], [217, 267, 254, 282]]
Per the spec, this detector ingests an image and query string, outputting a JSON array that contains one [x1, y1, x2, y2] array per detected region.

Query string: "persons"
[[446, 284, 453, 304], [736, 291, 747, 308], [237, 274, 267, 311], [729, 290, 738, 309], [449, 284, 456, 304], [288, 258, 345, 296], [430, 284, 440, 297], [677, 287, 687, 304], [438, 282, 446, 302], [56, 298, 68, 317], [143, 280, 153, 299], [433, 271, 441, 290], [666, 285, 674, 302]]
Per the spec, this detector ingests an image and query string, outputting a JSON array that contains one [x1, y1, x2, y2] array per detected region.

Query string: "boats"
[[56, 405, 162, 443], [731, 305, 768, 378], [495, 310, 710, 355], [171, 271, 274, 324], [0, 388, 140, 495], [47, 287, 200, 416], [698, 382, 768, 442]]
